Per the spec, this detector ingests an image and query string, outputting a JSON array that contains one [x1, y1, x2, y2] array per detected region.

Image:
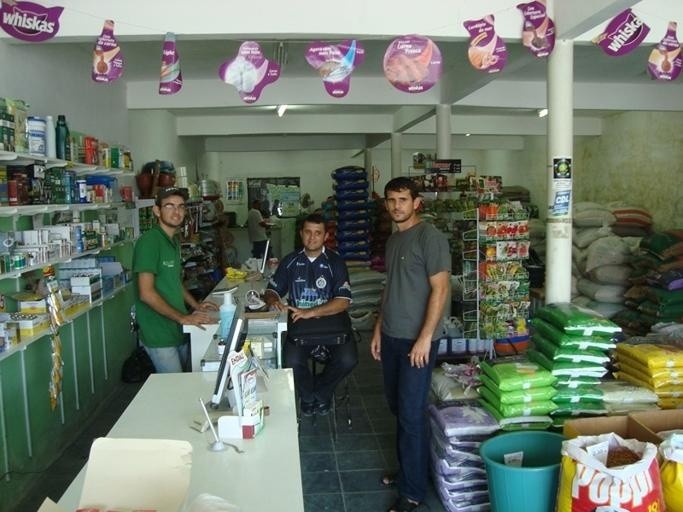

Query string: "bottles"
[[425, 154, 434, 174], [102, 144, 109, 169], [44, 114, 71, 161], [111, 144, 133, 171]]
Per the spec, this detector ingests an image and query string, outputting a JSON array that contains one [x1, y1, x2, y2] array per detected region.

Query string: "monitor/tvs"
[[255, 237, 271, 281], [188, 302, 269, 433]]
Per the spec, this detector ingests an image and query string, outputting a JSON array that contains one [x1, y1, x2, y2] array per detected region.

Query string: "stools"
[[311, 354, 358, 442]]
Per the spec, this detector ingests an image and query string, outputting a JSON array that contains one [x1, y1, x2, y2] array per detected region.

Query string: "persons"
[[248, 198, 281, 259], [131, 186, 221, 375], [369, 177, 452, 512], [261, 213, 358, 419]]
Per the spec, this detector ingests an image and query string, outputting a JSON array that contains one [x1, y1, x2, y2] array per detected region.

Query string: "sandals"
[[380, 475, 398, 487], [390, 497, 418, 512]]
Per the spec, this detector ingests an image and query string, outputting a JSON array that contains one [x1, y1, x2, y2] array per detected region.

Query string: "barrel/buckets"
[[479, 430, 567, 512], [56, 116, 70, 160]]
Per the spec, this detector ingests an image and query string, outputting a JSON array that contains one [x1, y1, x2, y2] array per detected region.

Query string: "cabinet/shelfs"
[[456, 201, 531, 358], [0, 152, 223, 511]]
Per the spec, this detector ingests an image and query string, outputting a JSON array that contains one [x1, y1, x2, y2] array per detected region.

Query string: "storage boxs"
[[561, 407, 682, 470]]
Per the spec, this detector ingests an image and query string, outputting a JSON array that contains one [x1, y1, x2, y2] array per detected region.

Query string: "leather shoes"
[[316, 399, 329, 415], [301, 400, 315, 416]]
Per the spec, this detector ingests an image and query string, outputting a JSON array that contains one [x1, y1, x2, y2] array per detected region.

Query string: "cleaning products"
[[44, 115, 58, 157]]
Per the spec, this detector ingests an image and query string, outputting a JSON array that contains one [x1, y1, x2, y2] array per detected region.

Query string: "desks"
[[54, 367, 304, 511]]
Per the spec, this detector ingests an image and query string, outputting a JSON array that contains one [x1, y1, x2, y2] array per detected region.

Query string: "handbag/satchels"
[[288, 310, 351, 345]]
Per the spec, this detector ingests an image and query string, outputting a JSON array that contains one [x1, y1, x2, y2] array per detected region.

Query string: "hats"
[[155, 188, 189, 206]]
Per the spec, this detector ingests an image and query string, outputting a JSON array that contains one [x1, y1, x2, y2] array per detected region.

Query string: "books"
[[237, 367, 258, 417]]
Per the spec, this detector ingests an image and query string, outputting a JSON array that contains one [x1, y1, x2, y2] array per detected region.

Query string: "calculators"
[[212, 285, 240, 295]]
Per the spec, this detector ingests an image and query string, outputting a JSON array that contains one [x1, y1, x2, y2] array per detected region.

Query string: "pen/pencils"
[[199, 397, 219, 442]]
